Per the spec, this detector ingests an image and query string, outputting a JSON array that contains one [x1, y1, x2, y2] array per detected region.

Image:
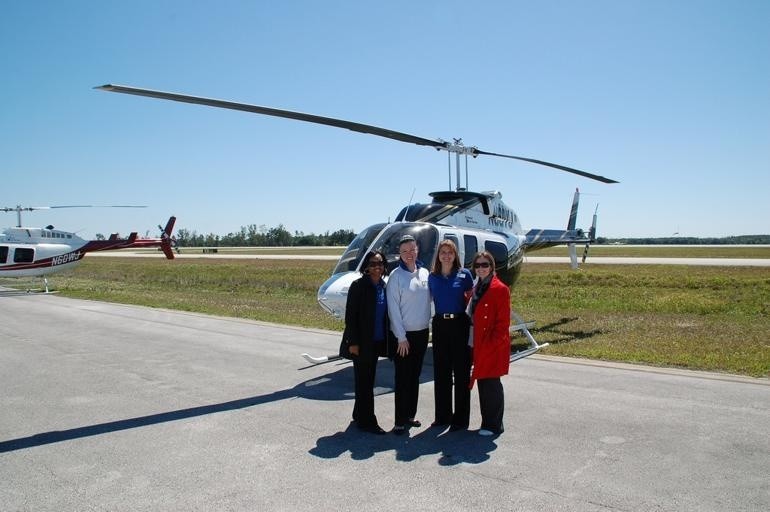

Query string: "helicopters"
[[1, 204, 178, 293], [90, 82, 620, 366]]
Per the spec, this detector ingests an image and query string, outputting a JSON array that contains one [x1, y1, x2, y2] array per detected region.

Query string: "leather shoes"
[[431, 421, 440, 427], [360, 424, 385, 435]]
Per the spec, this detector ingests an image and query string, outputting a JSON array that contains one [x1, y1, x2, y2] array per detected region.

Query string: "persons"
[[429, 240, 474, 434], [470, 250, 512, 436], [386, 232, 428, 435], [340, 250, 388, 435]]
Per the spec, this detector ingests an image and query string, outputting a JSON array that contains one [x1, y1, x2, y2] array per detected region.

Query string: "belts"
[[435, 312, 466, 320]]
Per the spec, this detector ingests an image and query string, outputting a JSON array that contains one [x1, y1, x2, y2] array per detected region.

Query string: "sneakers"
[[478, 429, 494, 436], [409, 420, 421, 427], [394, 425, 407, 434]]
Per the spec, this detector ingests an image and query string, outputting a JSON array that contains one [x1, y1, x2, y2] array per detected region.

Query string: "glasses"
[[399, 248, 417, 254], [474, 263, 491, 268], [367, 261, 385, 267]]
